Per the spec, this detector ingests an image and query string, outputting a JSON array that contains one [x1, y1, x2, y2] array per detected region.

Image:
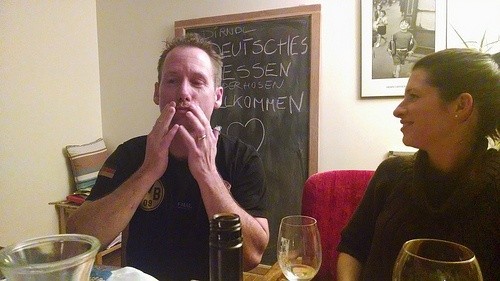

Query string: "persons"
[[335, 49, 500, 281], [373, 0, 392, 48], [65, 33, 269, 281], [387, 19, 417, 78]]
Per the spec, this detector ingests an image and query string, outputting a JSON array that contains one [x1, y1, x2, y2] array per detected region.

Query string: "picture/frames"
[[360, 0, 449, 99]]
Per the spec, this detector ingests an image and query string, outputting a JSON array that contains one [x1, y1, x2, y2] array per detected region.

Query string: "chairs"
[[300, 168, 377, 281]]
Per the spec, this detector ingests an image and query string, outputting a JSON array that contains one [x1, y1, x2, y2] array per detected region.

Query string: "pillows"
[[64, 137, 110, 191]]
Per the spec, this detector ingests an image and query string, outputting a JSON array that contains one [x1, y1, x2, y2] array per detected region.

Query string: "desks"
[[47, 199, 123, 265]]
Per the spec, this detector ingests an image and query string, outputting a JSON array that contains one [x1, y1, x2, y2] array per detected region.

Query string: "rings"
[[198, 135, 206, 140]]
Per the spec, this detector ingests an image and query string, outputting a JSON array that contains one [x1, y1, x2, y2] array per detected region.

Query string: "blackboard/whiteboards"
[[175, 4, 321, 267]]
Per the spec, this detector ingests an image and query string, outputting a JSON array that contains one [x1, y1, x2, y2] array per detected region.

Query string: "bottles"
[[209, 212, 243, 281]]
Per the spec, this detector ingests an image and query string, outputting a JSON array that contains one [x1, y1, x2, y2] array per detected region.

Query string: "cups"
[[0, 234, 101, 281], [391, 238, 483, 281], [277, 215, 322, 281]]
